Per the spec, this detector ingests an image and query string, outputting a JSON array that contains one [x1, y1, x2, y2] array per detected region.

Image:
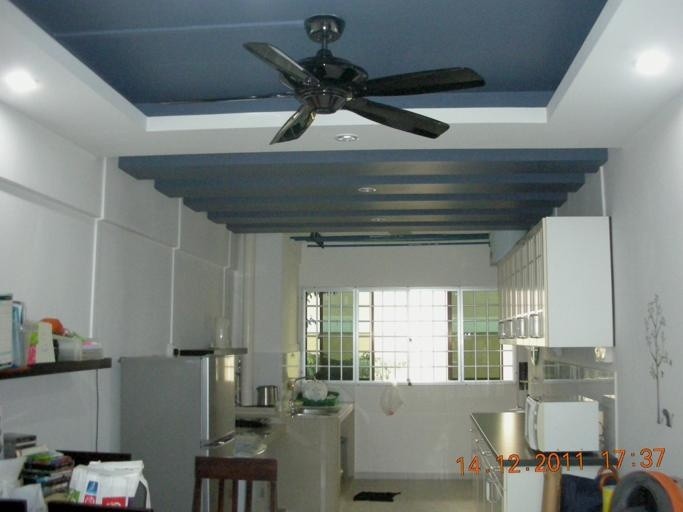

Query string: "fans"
[[160, 15, 485, 145]]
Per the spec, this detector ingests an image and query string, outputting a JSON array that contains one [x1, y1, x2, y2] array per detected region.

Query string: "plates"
[[305, 380, 328, 402]]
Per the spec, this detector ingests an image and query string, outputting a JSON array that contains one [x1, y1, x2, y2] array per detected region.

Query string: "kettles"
[[256, 385, 278, 407]]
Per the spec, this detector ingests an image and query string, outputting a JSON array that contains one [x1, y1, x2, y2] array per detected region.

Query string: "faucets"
[[289, 375, 316, 415]]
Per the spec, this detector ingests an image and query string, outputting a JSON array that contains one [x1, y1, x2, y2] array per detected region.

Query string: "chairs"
[[188, 456, 278, 511]]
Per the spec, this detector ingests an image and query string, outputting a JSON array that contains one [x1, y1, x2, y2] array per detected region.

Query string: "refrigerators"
[[115, 354, 241, 512]]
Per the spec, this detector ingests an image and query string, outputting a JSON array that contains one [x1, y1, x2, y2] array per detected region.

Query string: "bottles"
[[280, 380, 296, 424]]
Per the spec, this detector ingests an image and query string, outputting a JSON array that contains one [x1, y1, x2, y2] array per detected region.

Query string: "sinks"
[[291, 406, 340, 417]]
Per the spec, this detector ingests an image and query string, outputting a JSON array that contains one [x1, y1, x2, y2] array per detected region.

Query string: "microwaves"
[[524, 392, 600, 453]]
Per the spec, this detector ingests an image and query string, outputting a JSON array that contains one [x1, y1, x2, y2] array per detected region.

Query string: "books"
[[0, 294, 27, 371], [17, 450, 74, 498]]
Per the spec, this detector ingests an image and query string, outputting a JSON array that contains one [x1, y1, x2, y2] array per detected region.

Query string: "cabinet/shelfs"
[[497, 215, 613, 346], [470, 418, 504, 511]]
